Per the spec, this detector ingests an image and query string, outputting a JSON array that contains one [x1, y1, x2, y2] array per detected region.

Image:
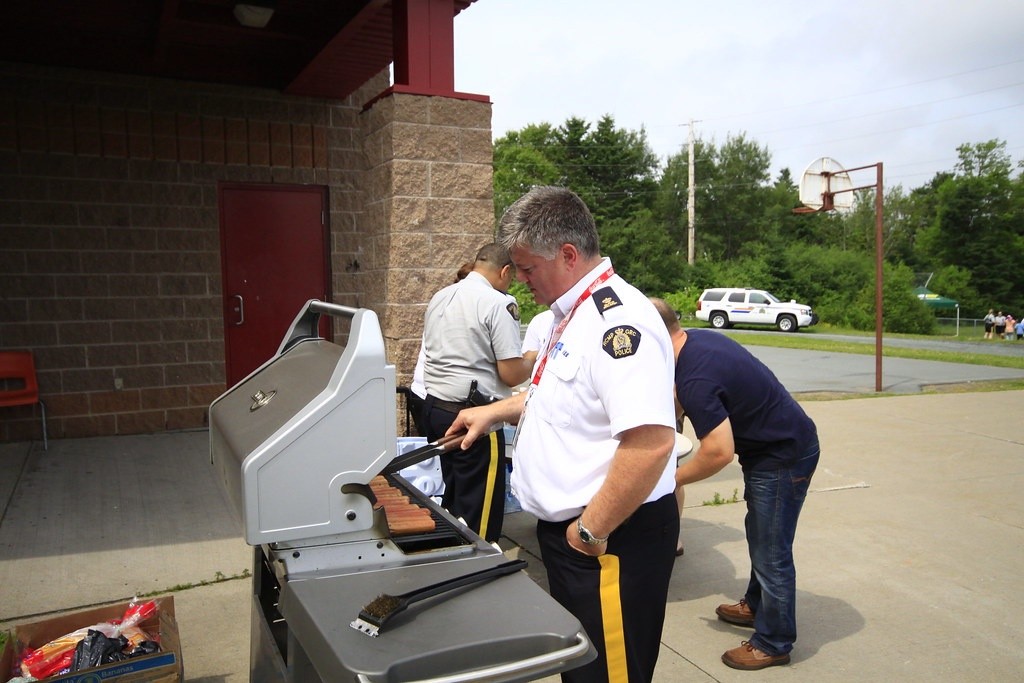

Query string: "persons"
[[995, 311, 1007, 341], [1014, 319, 1024, 340], [424, 243, 539, 544], [406, 258, 478, 439], [983, 308, 995, 339], [1004, 314, 1016, 341], [648, 296, 820, 671], [443, 184, 681, 683], [521, 309, 555, 378]]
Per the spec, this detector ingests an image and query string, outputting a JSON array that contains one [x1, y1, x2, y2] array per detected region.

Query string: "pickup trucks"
[[696, 287, 813, 332]]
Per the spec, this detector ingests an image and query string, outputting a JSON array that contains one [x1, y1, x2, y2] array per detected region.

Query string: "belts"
[[425, 394, 466, 414]]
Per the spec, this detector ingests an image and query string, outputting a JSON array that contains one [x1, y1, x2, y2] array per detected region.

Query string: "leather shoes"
[[716, 601, 757, 625], [722, 642, 792, 670]]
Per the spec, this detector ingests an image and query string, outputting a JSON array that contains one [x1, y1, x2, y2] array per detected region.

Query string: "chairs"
[[0, 350, 49, 450]]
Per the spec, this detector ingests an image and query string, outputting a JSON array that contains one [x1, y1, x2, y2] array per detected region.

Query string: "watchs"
[[578, 517, 608, 545]]
